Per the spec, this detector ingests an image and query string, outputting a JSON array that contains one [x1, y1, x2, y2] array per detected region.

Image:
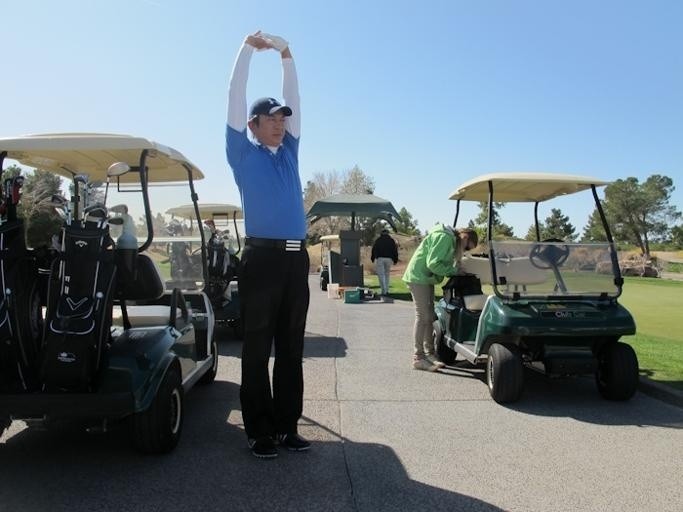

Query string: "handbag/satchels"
[[441, 272, 484, 307]]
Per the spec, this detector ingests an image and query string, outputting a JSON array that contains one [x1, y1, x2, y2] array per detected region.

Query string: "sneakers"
[[246, 431, 310, 458], [412, 354, 445, 372]]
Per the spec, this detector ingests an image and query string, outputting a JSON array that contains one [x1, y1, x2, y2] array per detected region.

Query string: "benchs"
[[455, 256, 550, 312], [29, 247, 193, 328]]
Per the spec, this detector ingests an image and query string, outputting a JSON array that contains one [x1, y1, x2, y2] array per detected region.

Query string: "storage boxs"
[[344, 288, 360, 303]]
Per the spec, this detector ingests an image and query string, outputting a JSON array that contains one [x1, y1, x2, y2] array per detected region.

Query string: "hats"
[[248, 97, 292, 116]]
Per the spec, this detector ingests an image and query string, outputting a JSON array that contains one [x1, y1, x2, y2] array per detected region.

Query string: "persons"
[[370, 229, 397, 295], [225, 29, 312, 457], [401, 221, 478, 372]]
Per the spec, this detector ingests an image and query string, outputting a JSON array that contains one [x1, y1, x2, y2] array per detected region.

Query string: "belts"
[[246, 237, 306, 252]]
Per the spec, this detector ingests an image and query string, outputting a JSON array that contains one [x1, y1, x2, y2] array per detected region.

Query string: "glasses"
[[464, 240, 470, 251]]
